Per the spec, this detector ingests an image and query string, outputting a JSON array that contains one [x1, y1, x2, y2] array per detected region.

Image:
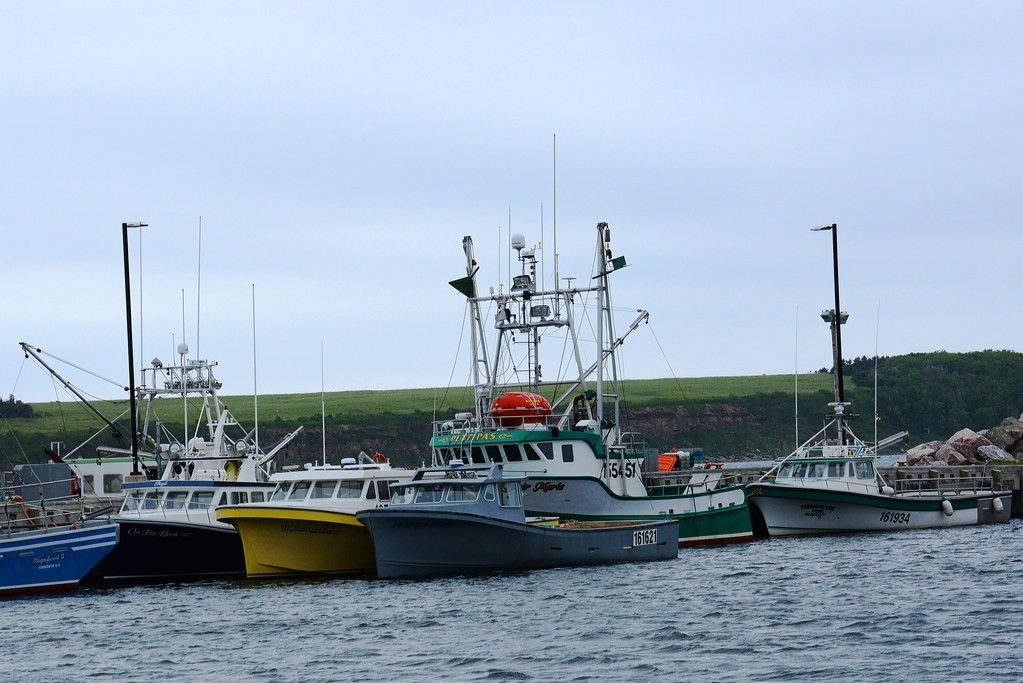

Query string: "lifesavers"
[[372, 453, 386, 463]]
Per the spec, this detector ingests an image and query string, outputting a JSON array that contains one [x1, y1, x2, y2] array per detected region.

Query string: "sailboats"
[[0, 134, 754, 603], [747, 222, 1014, 539]]
